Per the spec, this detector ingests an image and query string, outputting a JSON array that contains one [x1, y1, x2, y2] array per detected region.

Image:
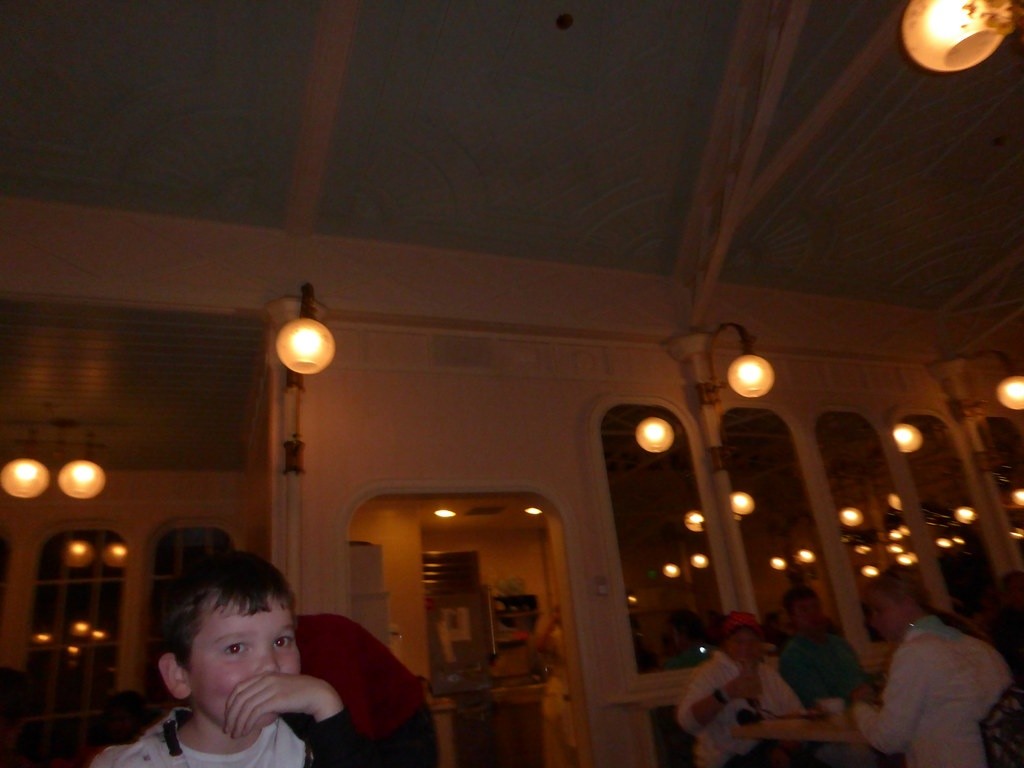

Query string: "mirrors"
[[584, 393, 1024, 709]]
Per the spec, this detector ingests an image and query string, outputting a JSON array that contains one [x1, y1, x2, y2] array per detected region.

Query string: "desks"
[[728, 710, 875, 756]]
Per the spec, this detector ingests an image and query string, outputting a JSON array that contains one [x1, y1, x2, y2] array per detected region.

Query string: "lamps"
[[924, 348, 1024, 411], [896, 0, 1024, 76], [710, 319, 777, 404], [31, 535, 129, 662], [0, 445, 106, 502], [274, 284, 342, 377]]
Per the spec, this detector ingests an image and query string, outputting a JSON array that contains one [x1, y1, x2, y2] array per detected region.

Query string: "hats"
[[723, 611, 759, 633]]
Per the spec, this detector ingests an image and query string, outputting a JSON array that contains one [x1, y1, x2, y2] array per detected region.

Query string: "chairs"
[[973, 682, 1024, 768], [649, 701, 697, 768]]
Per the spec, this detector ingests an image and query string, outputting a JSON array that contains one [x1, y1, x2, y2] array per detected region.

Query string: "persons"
[[628, 564, 1024, 768], [288, 613, 442, 768], [51, 550, 363, 768]]
